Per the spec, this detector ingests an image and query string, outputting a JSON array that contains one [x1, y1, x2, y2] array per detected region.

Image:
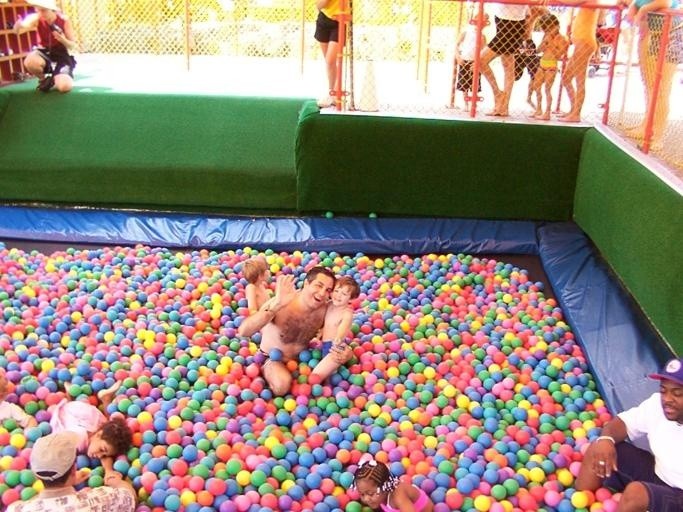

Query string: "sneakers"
[[36, 75, 54, 91]]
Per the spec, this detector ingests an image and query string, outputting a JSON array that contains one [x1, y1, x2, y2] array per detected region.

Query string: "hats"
[[647, 358, 683, 387], [27, 1, 59, 11]]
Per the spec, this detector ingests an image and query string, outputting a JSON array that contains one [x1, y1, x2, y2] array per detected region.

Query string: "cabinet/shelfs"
[[0, 0, 64, 89]]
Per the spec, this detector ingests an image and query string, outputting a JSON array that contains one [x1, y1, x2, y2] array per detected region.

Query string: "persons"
[[312, 0, 353, 107], [457, 2, 606, 124], [623, 0, 683, 149], [578, 359, 683, 512], [13, 0, 77, 93]]
